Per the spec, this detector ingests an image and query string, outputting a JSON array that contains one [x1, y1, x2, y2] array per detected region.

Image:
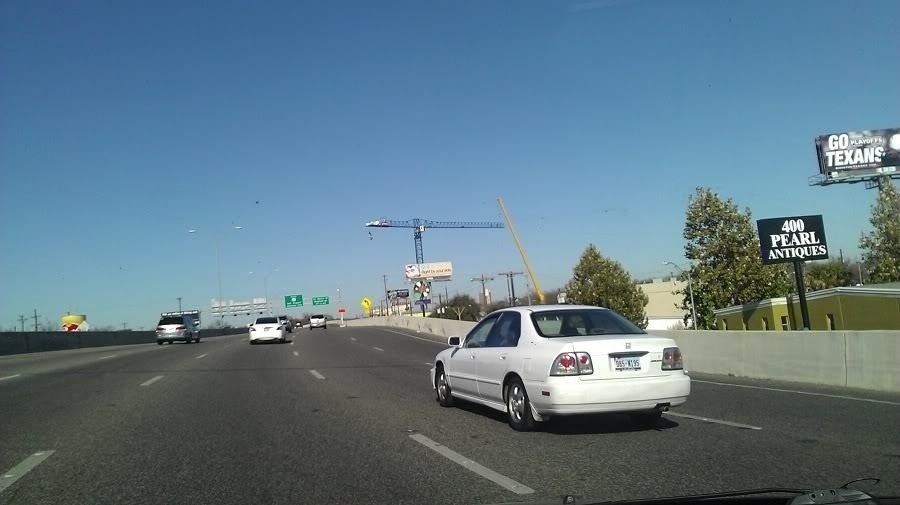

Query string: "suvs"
[[155, 310, 202, 347]]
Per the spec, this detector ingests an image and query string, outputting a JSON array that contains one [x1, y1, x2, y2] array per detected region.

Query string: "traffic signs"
[[311, 295, 330, 306], [284, 294, 304, 308]]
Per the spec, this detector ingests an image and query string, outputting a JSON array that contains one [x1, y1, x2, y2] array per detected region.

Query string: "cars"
[[246, 312, 327, 345], [422, 303, 696, 434]]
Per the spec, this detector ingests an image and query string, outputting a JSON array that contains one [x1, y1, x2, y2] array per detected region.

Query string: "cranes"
[[469, 271, 526, 307], [361, 217, 506, 266]]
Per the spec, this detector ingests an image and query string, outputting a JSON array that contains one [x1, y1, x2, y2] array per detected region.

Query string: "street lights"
[[661, 259, 698, 330], [186, 226, 245, 329], [175, 297, 183, 312], [247, 268, 280, 311]]
